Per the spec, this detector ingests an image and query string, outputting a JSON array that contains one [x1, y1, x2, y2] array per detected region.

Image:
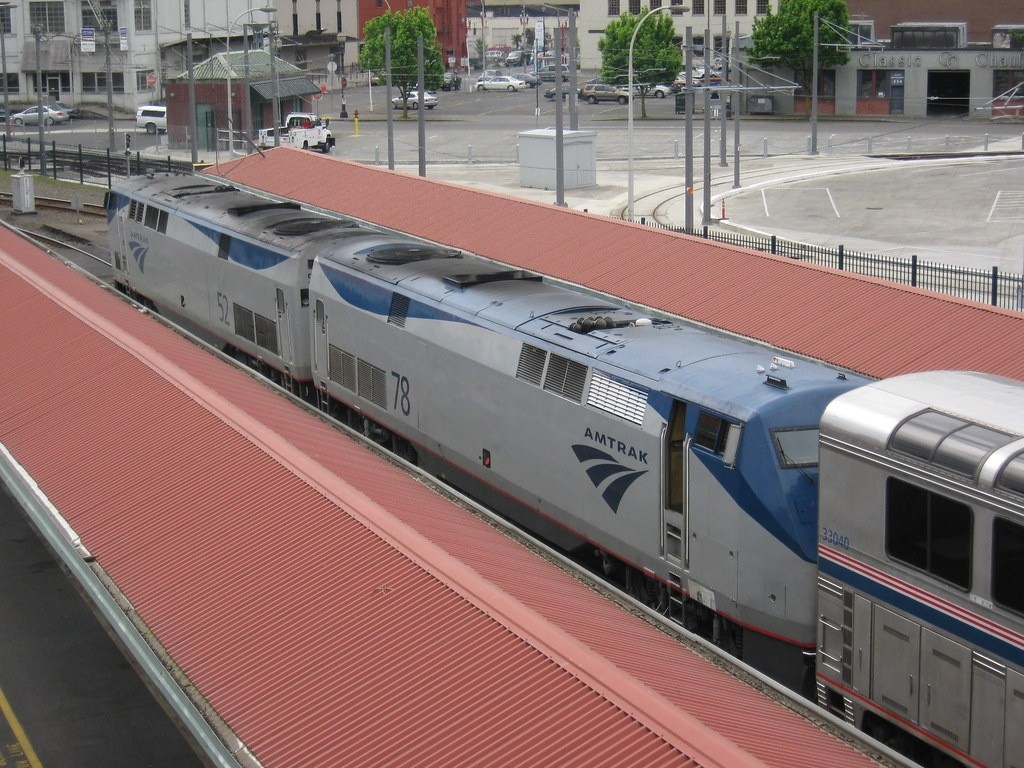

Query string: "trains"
[[101, 169, 1024, 768]]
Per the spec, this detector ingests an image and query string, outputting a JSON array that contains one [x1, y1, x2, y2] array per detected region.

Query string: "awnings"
[[250, 76, 322, 100]]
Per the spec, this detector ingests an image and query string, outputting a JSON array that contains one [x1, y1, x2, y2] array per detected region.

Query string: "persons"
[[303, 117, 312, 128]]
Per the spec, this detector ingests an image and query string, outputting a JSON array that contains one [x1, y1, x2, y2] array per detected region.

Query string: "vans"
[[135, 106, 167, 134]]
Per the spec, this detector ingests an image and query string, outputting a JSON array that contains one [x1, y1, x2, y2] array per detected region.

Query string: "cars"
[[544, 71, 700, 104], [9, 101, 81, 126], [391, 91, 439, 111], [475, 64, 569, 92], [484, 44, 524, 66]]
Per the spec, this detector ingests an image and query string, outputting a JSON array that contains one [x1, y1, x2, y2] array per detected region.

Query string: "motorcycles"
[[442, 72, 453, 91]]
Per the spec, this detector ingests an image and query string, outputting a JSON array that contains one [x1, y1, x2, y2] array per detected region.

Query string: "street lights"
[[627, 4, 690, 221], [226, 6, 279, 160]]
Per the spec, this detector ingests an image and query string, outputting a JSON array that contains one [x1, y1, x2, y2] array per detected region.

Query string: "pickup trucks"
[[258, 112, 335, 152]]
